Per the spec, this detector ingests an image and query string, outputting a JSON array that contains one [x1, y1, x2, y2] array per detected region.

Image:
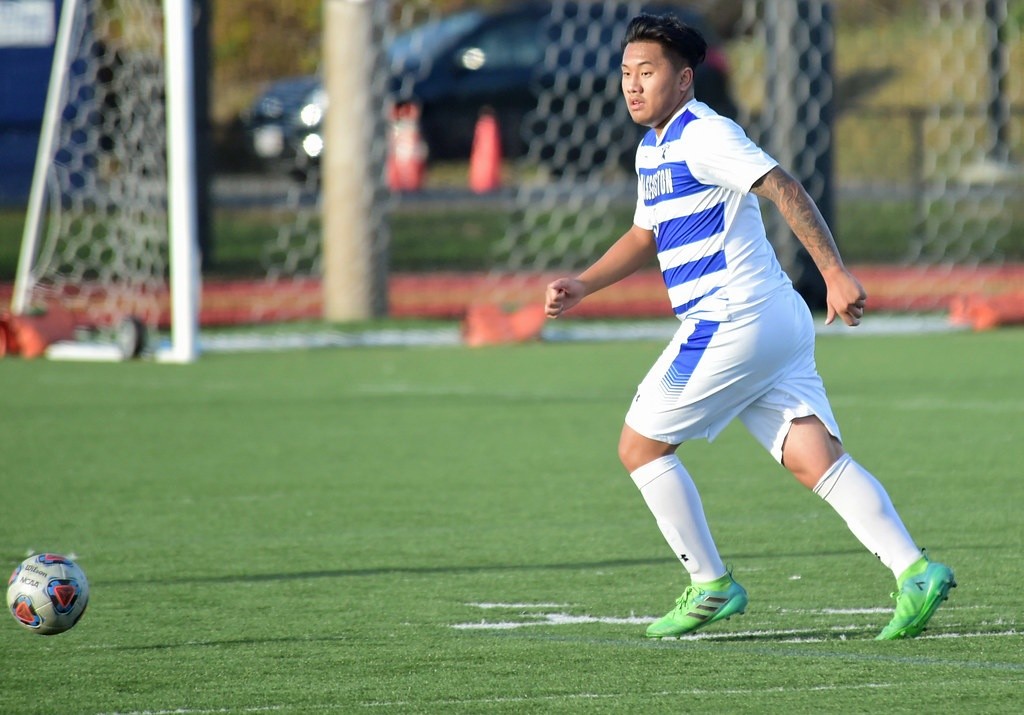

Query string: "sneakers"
[[644, 562, 746, 639], [874, 548, 957, 640]]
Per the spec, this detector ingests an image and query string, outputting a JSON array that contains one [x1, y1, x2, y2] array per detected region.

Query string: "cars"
[[248, 0, 735, 183]]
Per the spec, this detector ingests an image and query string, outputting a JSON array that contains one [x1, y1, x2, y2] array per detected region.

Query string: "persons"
[[544, 14, 957, 641]]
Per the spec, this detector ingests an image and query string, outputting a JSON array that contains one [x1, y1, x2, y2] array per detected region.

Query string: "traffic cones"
[[471, 114, 503, 193], [384, 101, 431, 198]]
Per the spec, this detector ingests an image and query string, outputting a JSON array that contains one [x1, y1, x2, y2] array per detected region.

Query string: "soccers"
[[6, 552, 91, 635]]
